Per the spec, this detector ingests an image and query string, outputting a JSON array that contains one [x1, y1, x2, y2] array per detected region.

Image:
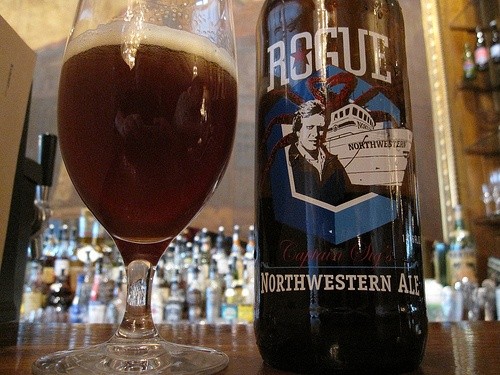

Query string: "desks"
[[0, 321, 500, 375]]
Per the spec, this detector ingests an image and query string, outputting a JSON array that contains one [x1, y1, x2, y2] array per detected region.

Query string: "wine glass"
[[34, 0, 239, 374]]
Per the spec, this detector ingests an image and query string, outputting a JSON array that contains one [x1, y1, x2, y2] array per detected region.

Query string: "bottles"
[[473, 25, 489, 72], [150, 223, 254, 324], [434, 243, 447, 285], [446, 204, 481, 287], [490, 171, 500, 215], [450, 274, 500, 322], [489, 19, 500, 64], [463, 41, 478, 81], [20, 206, 127, 325], [255, 0, 429, 375], [481, 183, 495, 217]]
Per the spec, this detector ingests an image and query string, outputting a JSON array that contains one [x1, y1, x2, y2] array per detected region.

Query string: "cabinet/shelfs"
[[450, 0, 500, 319]]
